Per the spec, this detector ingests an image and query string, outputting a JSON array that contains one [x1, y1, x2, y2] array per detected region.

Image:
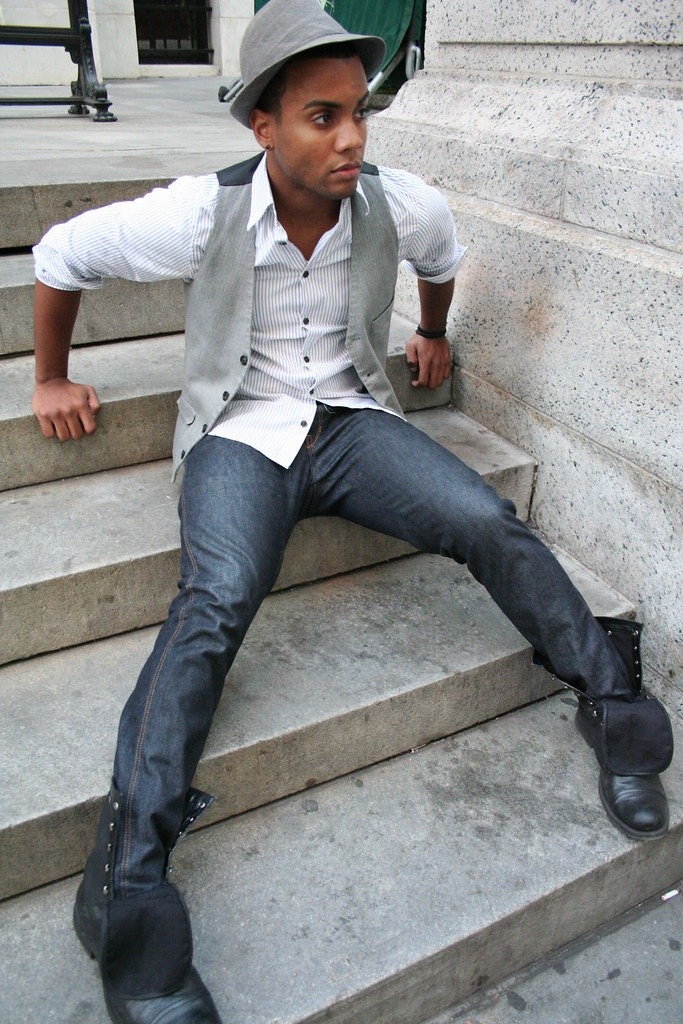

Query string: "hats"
[[229, 0, 386, 130]]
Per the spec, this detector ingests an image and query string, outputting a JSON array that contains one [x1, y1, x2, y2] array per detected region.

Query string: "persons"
[[28, 0, 674, 1024]]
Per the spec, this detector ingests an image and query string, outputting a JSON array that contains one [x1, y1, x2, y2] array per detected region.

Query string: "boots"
[[73, 774, 225, 1024], [531, 617, 670, 845]]
